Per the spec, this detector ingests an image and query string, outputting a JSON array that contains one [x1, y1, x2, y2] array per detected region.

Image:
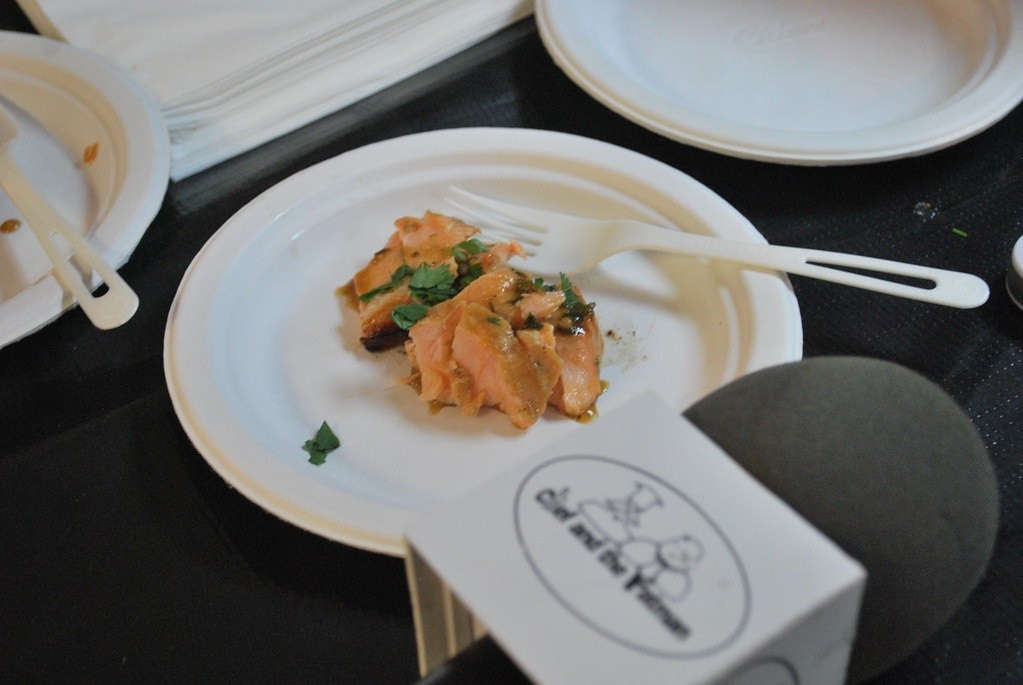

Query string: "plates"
[[0, 30, 169, 351], [164, 126, 804, 560], [535, 0, 1023, 167]]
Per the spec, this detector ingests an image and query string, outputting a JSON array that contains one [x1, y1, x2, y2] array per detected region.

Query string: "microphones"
[[398, 354, 1005, 685]]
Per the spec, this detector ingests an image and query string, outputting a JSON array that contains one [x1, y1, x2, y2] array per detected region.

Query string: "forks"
[[444, 183, 990, 309]]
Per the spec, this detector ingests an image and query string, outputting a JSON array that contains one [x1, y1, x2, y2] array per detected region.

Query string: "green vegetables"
[[302, 238, 596, 466]]
